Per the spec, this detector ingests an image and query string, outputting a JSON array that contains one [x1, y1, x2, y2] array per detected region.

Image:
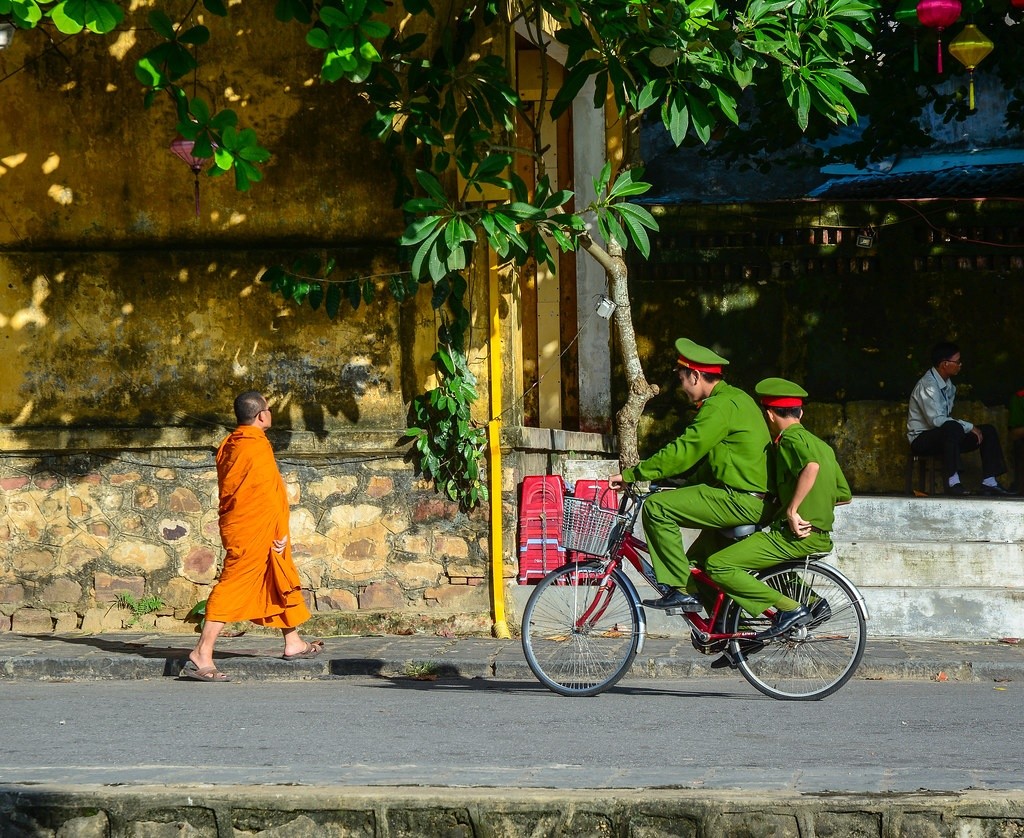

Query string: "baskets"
[[561, 495, 627, 556]]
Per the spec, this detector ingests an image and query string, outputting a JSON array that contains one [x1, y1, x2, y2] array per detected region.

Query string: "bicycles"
[[522, 480, 869, 702]]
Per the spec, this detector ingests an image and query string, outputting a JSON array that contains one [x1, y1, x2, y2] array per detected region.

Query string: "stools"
[[905, 453, 953, 495], [517, 475, 620, 589]]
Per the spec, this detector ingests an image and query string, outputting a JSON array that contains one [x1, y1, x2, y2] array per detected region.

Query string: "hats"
[[673, 337, 730, 374], [755, 377, 808, 407]]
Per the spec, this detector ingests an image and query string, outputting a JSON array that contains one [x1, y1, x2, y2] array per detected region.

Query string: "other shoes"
[[947, 483, 970, 495], [981, 483, 1015, 495]]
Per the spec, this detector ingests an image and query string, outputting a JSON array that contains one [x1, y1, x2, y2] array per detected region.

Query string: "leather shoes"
[[807, 598, 831, 628], [642, 588, 703, 612], [711, 640, 764, 668], [756, 603, 813, 641]]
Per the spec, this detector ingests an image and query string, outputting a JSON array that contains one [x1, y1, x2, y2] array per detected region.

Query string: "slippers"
[[183, 666, 230, 682], [282, 640, 326, 661]]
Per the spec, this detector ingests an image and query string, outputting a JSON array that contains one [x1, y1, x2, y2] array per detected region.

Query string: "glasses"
[[942, 360, 961, 365], [254, 407, 272, 417]]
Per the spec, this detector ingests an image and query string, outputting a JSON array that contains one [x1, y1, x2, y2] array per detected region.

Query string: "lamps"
[[856, 235, 873, 249]]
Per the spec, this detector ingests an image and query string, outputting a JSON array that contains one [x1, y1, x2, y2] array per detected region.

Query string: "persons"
[[906, 342, 1020, 497], [608, 337, 782, 668], [189, 391, 323, 680], [705, 377, 852, 641]]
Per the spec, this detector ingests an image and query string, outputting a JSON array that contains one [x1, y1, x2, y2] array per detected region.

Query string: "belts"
[[780, 519, 828, 535], [751, 491, 772, 501]]
